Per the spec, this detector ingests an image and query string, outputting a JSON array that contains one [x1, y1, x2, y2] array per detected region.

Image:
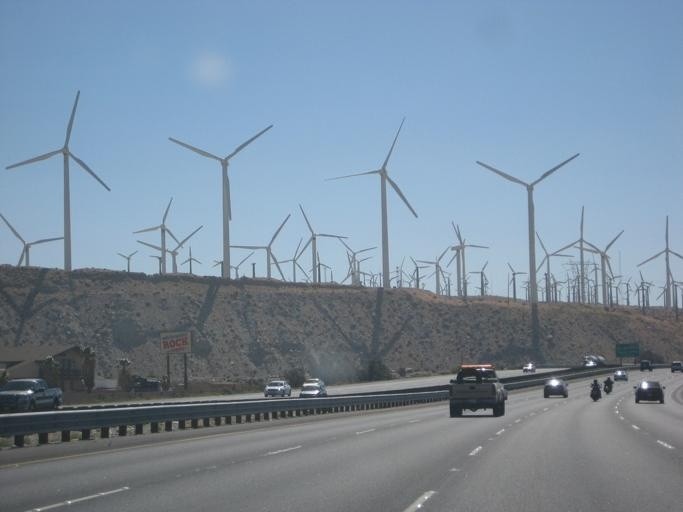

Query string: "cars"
[[522, 362, 568, 398], [631, 380, 666, 404], [614, 359, 683, 380], [263, 378, 327, 399]]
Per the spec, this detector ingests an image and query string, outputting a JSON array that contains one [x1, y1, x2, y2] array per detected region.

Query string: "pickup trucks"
[[0, 377, 64, 414], [446, 363, 507, 417]]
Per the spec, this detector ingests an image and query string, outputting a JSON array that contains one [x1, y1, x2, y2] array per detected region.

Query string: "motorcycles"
[[589, 375, 614, 402]]
